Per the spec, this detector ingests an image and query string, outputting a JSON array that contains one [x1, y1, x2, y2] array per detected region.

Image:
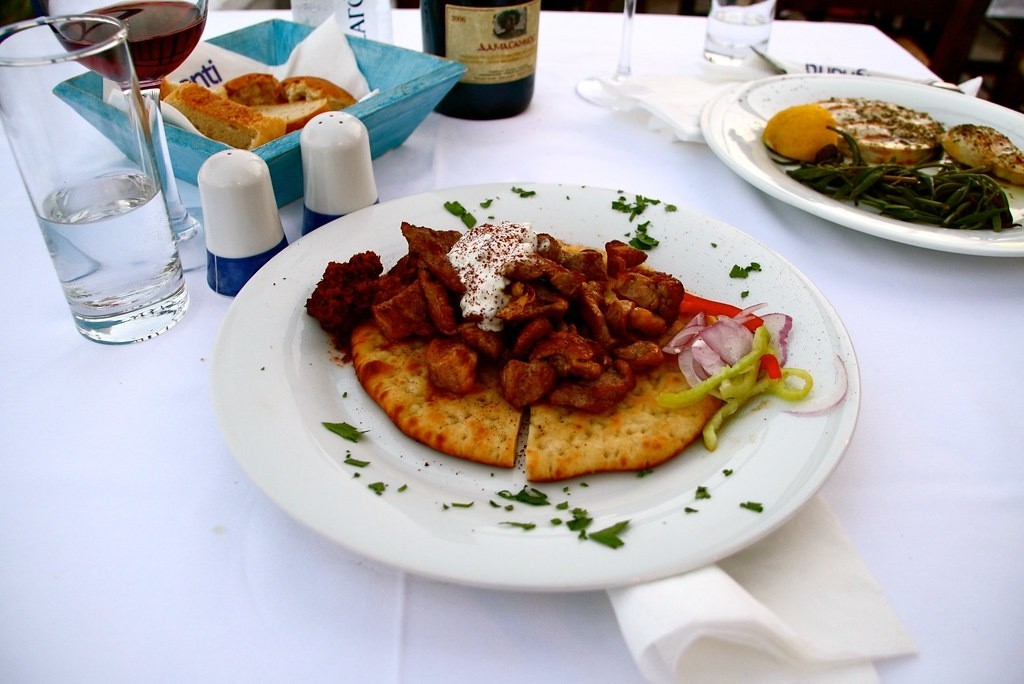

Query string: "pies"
[[526, 238, 754, 483], [351, 319, 523, 467]]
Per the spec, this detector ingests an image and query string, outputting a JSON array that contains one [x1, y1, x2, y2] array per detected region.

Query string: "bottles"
[[418, 0, 541, 120], [289, 0, 394, 45], [300, 108, 382, 238], [196, 147, 290, 300]]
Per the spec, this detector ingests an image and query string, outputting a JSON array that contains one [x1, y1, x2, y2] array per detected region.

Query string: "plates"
[[700, 72, 1024, 259], [210, 182, 862, 594], [54, 17, 468, 209]]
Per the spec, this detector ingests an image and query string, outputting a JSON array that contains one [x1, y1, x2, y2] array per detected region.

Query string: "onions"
[[660, 301, 848, 415]]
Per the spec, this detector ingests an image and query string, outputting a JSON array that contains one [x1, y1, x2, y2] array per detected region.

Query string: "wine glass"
[[573, 0, 656, 111], [40, 0, 209, 275]]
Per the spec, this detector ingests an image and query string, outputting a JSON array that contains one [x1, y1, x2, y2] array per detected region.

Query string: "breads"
[[160, 74, 358, 150]]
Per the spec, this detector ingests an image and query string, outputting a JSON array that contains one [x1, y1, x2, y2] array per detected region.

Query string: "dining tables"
[[0, 8, 1024, 684]]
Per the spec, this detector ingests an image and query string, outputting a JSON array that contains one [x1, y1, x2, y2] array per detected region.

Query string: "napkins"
[[607, 64, 755, 144], [101, 10, 383, 137], [603, 492, 919, 684]]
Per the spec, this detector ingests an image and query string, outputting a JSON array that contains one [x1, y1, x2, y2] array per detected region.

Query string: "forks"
[[748, 45, 793, 74]]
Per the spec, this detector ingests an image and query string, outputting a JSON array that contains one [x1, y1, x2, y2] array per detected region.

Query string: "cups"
[[0, 14, 189, 345], [703, 0, 777, 82]]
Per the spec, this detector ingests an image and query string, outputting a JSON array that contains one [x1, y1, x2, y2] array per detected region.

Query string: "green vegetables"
[[770, 126, 1022, 232]]
